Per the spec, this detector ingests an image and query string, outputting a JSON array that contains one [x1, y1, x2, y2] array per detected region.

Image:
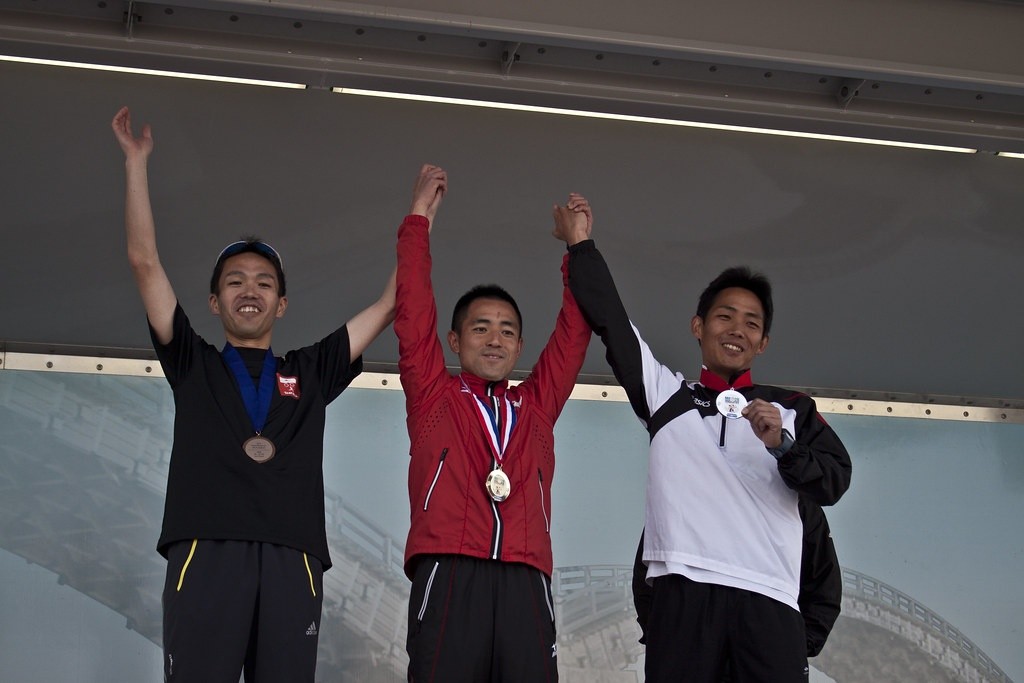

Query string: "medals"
[[486, 463, 510, 501], [243, 431, 275, 464], [716, 386, 748, 419]]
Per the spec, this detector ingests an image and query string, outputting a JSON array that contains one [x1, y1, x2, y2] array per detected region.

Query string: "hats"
[[213, 240, 283, 271]]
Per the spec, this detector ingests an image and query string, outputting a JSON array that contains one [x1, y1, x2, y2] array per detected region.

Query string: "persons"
[[110, 108, 445, 683], [394, 165, 593, 683], [553, 194, 852, 683]]
